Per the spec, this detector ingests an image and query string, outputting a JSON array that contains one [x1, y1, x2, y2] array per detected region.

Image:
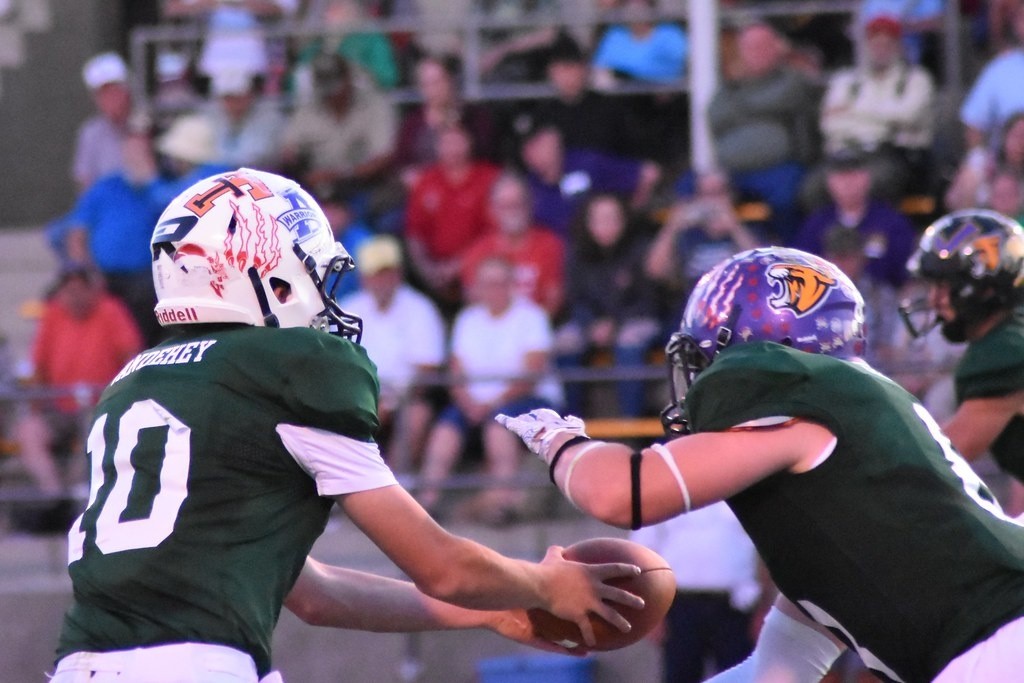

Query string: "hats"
[[207, 66, 254, 97], [355, 233, 401, 279], [80, 49, 128, 92], [824, 146, 867, 176], [867, 17, 902, 41]]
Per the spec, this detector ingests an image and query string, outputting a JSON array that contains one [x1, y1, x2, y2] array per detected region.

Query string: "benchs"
[[1, 2, 1021, 505]]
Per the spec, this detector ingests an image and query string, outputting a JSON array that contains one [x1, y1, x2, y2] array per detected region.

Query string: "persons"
[[3, 1, 1022, 524], [492, 243, 1024, 683], [46, 167, 647, 683]]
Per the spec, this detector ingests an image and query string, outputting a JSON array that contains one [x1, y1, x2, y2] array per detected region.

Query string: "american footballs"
[[529, 536, 675, 651]]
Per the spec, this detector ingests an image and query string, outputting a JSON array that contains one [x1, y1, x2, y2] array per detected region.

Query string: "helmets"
[[150, 166, 363, 347], [895, 205, 1024, 345], [658, 245, 866, 440]]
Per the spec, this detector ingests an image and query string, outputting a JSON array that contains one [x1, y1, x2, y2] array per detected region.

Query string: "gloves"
[[494, 408, 587, 465]]
